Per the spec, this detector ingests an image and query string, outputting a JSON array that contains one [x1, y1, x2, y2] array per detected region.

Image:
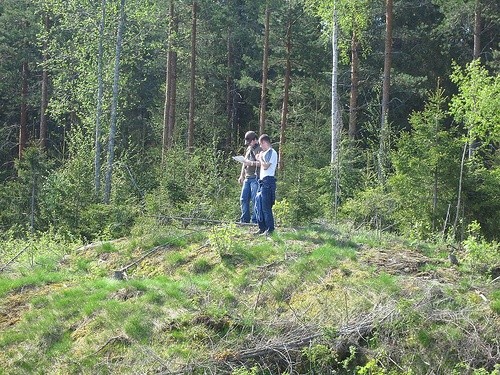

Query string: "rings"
[[239, 178, 241, 181]]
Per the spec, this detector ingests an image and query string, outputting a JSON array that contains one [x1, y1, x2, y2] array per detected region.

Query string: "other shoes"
[[259, 228, 270, 237], [253, 227, 267, 236]]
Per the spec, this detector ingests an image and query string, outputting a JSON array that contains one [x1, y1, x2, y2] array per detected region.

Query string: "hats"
[[245, 131, 255, 145]]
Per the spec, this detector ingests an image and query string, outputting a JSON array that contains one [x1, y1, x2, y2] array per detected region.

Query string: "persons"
[[238, 131, 263, 224], [252, 134, 277, 238]]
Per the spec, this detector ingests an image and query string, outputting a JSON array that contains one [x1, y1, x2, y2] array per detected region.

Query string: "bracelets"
[[250, 161, 254, 167]]
